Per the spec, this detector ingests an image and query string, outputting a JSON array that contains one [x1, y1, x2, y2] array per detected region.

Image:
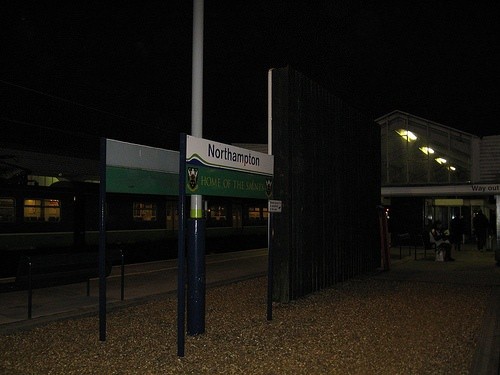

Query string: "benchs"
[[399, 232, 437, 261]]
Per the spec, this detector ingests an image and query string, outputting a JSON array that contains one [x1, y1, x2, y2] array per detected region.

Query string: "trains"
[[1, 146, 272, 284]]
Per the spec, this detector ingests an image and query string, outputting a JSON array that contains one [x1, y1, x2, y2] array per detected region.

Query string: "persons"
[[428, 219, 456, 262], [472, 208, 490, 252], [489, 208, 496, 241], [449, 212, 466, 251]]
